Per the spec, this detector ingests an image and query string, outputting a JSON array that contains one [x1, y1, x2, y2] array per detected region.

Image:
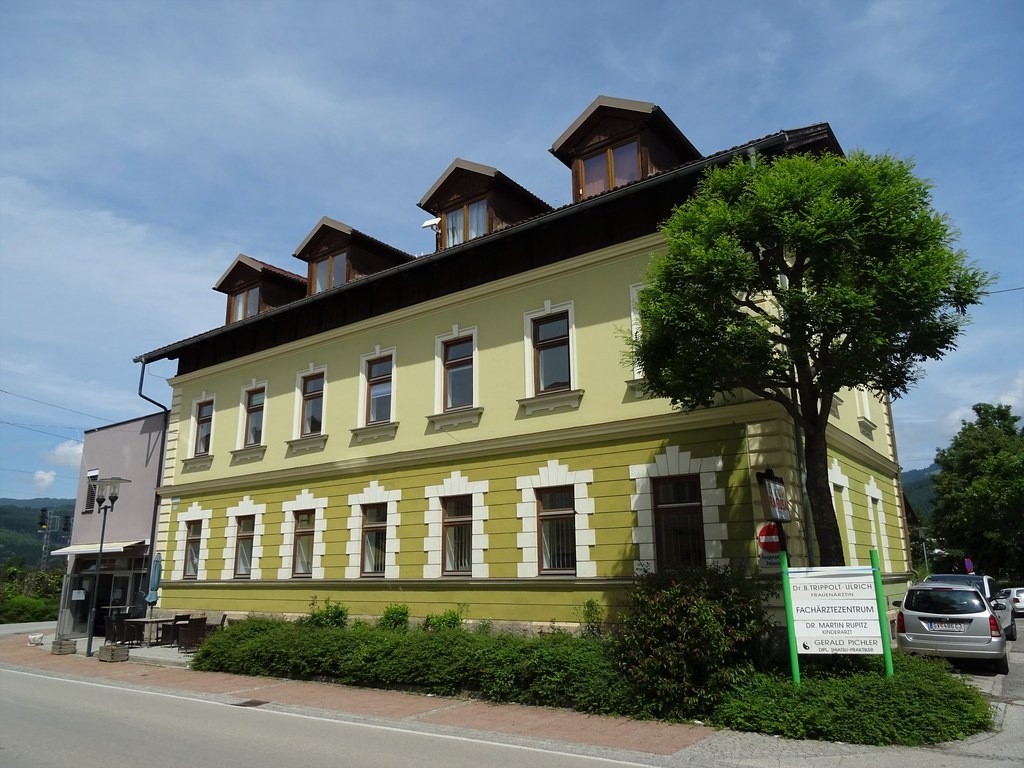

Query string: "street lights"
[[909, 526, 931, 573], [86, 475, 132, 659]]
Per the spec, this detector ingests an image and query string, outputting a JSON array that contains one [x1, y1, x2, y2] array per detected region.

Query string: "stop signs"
[[759, 524, 782, 554]]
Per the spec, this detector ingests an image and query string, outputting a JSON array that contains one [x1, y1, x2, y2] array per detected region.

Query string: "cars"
[[920, 572, 1018, 641], [1000, 587, 1024, 617], [891, 582, 1009, 674]]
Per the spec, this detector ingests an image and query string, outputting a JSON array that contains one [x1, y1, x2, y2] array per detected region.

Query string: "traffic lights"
[[37, 506, 47, 527], [61, 512, 72, 531]]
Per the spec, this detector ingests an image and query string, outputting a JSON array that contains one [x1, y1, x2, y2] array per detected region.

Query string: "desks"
[[124, 617, 176, 646], [101, 604, 137, 616]]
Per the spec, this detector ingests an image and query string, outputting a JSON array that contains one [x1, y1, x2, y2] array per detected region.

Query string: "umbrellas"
[[145, 553, 160, 647]]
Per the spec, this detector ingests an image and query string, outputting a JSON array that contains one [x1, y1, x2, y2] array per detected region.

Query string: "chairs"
[[104, 614, 227, 652]]
